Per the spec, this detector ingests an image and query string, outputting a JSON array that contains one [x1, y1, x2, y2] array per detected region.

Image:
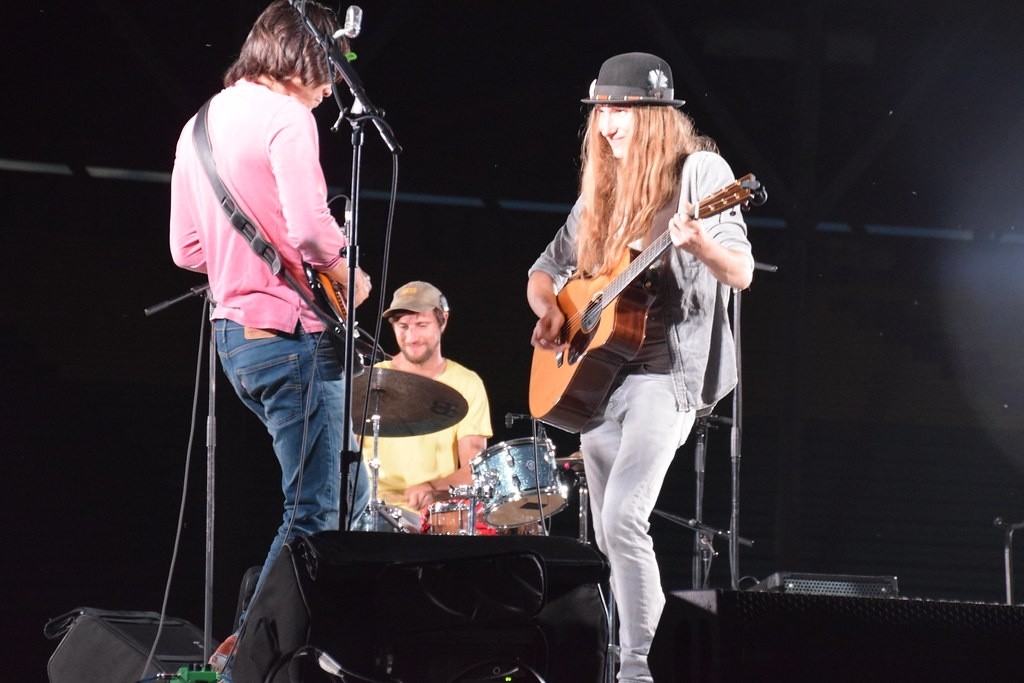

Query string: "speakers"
[[231, 530, 611, 683], [47, 608, 222, 683], [646, 584, 1024, 683], [746, 571, 898, 598]]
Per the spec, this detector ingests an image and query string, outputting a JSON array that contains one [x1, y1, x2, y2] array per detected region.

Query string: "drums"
[[353, 502, 402, 533], [426, 500, 507, 535], [469, 436, 569, 529]]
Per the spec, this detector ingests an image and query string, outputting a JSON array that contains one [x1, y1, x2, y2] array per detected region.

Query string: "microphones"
[[504, 414, 514, 429], [345, 200, 352, 241]]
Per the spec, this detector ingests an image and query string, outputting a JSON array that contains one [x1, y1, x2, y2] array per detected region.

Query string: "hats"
[[381, 280, 449, 318], [581, 53, 686, 107]]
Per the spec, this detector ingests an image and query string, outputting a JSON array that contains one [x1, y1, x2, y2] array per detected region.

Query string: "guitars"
[[526, 171, 768, 434], [305, 200, 365, 378]]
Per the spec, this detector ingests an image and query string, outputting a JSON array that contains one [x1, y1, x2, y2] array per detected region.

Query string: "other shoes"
[[208, 635, 237, 667]]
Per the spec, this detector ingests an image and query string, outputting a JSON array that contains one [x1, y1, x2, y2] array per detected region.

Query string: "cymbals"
[[554, 449, 585, 467], [348, 365, 469, 439]]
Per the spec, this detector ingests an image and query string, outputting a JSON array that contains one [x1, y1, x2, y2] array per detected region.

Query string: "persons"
[[169, 0, 372, 683], [526, 52, 754, 683], [353, 280, 493, 515]]
